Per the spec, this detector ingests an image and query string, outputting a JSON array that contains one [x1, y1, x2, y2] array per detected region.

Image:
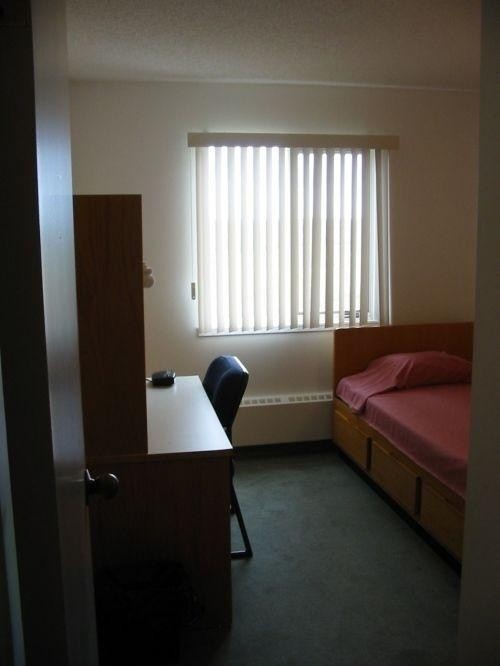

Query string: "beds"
[[330, 322, 473, 572]]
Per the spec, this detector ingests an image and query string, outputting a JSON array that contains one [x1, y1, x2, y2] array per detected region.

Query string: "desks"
[[86, 375, 238, 664]]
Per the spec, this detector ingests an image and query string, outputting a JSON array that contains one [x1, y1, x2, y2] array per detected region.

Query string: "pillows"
[[375, 349, 465, 391]]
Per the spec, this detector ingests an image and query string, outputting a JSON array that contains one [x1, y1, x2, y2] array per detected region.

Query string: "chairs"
[[202, 355, 254, 560]]
